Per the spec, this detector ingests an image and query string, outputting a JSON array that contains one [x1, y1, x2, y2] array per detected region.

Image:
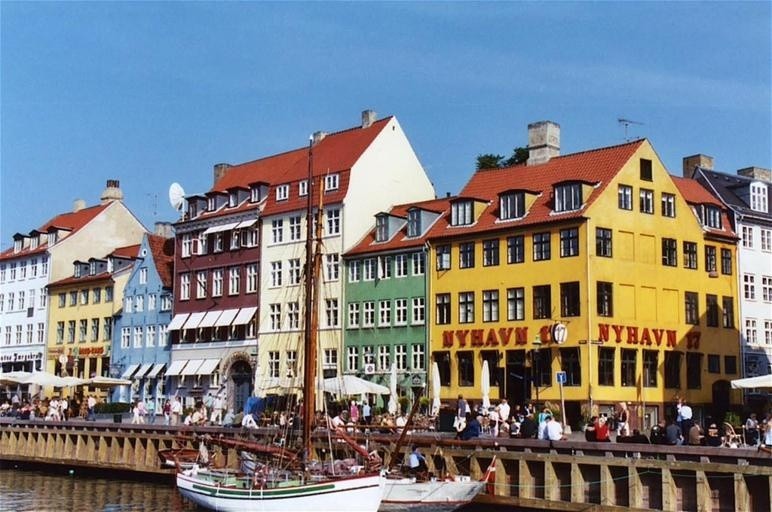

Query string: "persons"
[[745, 413, 771, 445], [511, 403, 567, 440], [454, 397, 510, 440], [674, 394, 693, 426], [1, 393, 98, 422], [132, 392, 413, 434], [584, 409, 649, 444], [408, 444, 430, 475], [649, 421, 740, 448]]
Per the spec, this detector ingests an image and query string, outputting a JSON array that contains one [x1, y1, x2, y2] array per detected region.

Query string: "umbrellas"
[[387, 360, 399, 415], [480, 357, 490, 415], [430, 359, 442, 416], [256, 370, 304, 393], [324, 373, 390, 400], [0, 369, 134, 387]]
